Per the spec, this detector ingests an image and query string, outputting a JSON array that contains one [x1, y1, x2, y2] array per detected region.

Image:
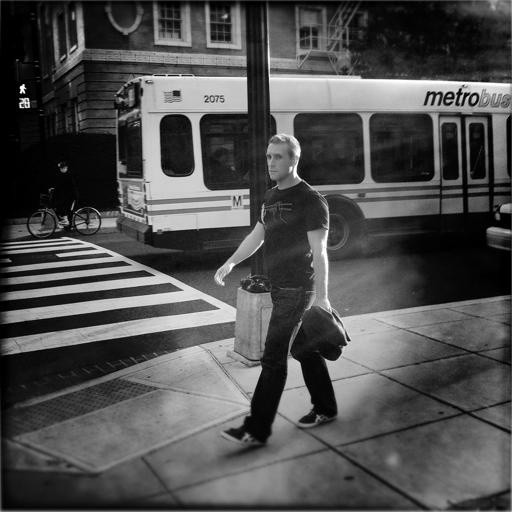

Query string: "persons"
[[211, 134, 337, 446], [47, 161, 77, 234]]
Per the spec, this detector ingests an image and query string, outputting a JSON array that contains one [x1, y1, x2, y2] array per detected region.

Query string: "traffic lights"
[[17, 82, 39, 110]]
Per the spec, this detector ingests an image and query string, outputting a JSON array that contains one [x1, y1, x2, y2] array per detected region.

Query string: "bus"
[[112, 73, 510, 262]]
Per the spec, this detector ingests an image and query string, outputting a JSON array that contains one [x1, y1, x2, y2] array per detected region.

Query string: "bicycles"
[[26, 190, 102, 239]]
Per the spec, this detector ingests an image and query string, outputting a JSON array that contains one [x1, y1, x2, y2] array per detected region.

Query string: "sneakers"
[[222, 428, 265, 446], [298, 410, 336, 428]]
